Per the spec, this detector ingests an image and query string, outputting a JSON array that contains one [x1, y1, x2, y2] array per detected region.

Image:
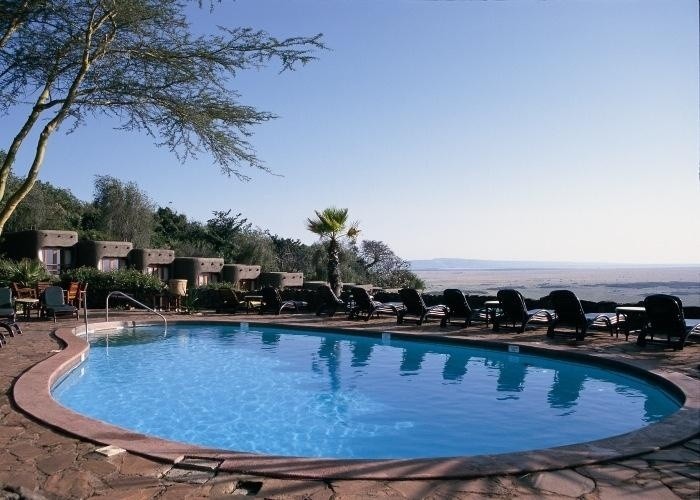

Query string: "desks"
[[484, 301, 506, 328], [614, 306, 654, 340], [425, 305, 450, 323]]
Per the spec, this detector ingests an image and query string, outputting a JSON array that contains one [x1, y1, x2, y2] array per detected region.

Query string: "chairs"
[[348, 288, 402, 321], [0, 281, 88, 347], [376, 304, 407, 322], [442, 289, 491, 327], [219, 285, 361, 317], [398, 288, 437, 325], [635, 293, 699, 352], [546, 289, 627, 342], [491, 289, 558, 334]]
